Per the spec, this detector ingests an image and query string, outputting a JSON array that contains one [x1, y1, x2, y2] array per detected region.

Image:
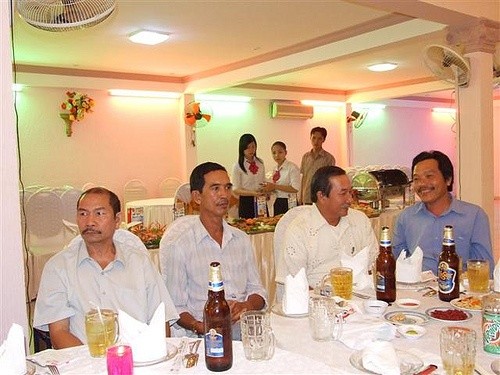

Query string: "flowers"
[[61, 92, 100, 122]]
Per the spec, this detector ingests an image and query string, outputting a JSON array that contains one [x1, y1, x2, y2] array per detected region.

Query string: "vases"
[[60, 111, 75, 137]]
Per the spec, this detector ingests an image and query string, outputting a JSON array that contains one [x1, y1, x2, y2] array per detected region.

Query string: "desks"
[[151, 203, 407, 291], [270, 274, 499, 375], [25, 328, 350, 375], [124, 197, 176, 225]]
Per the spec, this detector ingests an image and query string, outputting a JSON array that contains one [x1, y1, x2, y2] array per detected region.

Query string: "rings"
[[265, 183, 267, 185]]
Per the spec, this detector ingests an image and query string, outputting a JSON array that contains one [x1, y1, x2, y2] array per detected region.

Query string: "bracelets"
[[192, 320, 202, 335]]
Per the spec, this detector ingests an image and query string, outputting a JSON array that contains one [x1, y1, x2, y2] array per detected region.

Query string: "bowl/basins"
[[362, 300, 389, 313]]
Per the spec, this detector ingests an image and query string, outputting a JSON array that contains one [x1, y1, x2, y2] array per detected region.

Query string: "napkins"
[[339, 246, 374, 290], [114, 302, 173, 363], [280, 267, 309, 318], [395, 246, 424, 285]]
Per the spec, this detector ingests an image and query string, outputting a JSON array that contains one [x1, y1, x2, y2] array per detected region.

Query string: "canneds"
[[482, 309, 500, 356]]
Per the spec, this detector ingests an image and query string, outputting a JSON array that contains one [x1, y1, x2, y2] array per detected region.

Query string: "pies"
[[455, 296, 482, 309]]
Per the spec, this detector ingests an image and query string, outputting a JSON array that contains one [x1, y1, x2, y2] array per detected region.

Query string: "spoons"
[[423, 289, 439, 297], [186, 340, 202, 368]]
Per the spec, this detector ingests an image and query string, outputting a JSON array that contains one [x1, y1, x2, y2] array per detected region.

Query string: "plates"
[[349, 347, 424, 375], [491, 356, 500, 374], [26, 362, 36, 375], [398, 324, 427, 339], [271, 303, 309, 317], [383, 310, 430, 326], [425, 306, 472, 323], [451, 297, 481, 312], [395, 271, 494, 294], [133, 343, 178, 368], [395, 298, 421, 310]]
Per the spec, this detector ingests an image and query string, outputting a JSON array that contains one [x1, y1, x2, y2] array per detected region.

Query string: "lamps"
[[130, 29, 170, 46], [369, 60, 398, 73]]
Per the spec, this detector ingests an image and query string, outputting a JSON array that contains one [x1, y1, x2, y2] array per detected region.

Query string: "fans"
[[185, 100, 213, 146], [347, 110, 368, 129], [17, 0, 117, 31], [424, 40, 472, 86]]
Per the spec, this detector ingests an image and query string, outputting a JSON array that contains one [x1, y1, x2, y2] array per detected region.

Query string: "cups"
[[240, 310, 274, 361], [439, 327, 476, 375], [85, 306, 118, 357], [467, 259, 490, 290], [370, 202, 379, 209], [309, 298, 344, 342], [106, 346, 133, 375], [321, 266, 354, 299], [458, 256, 463, 284]]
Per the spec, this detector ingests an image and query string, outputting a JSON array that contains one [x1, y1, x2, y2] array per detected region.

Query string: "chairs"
[[18, 180, 190, 306]]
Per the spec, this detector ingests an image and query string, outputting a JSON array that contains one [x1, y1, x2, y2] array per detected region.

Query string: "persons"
[[299, 127, 335, 205], [387, 150, 495, 279], [259, 142, 301, 218], [283, 166, 381, 292], [31, 187, 181, 349], [232, 134, 270, 220], [167, 161, 271, 338]]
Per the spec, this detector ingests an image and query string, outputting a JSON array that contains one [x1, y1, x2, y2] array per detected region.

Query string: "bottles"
[[375, 226, 396, 301], [437, 225, 459, 299], [203, 262, 234, 371], [482, 291, 500, 353]]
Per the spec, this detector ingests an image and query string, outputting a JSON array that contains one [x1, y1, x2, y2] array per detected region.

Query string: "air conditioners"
[[270, 102, 314, 119]]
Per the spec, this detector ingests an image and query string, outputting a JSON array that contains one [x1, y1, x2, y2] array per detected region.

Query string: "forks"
[[27, 358, 59, 375]]
